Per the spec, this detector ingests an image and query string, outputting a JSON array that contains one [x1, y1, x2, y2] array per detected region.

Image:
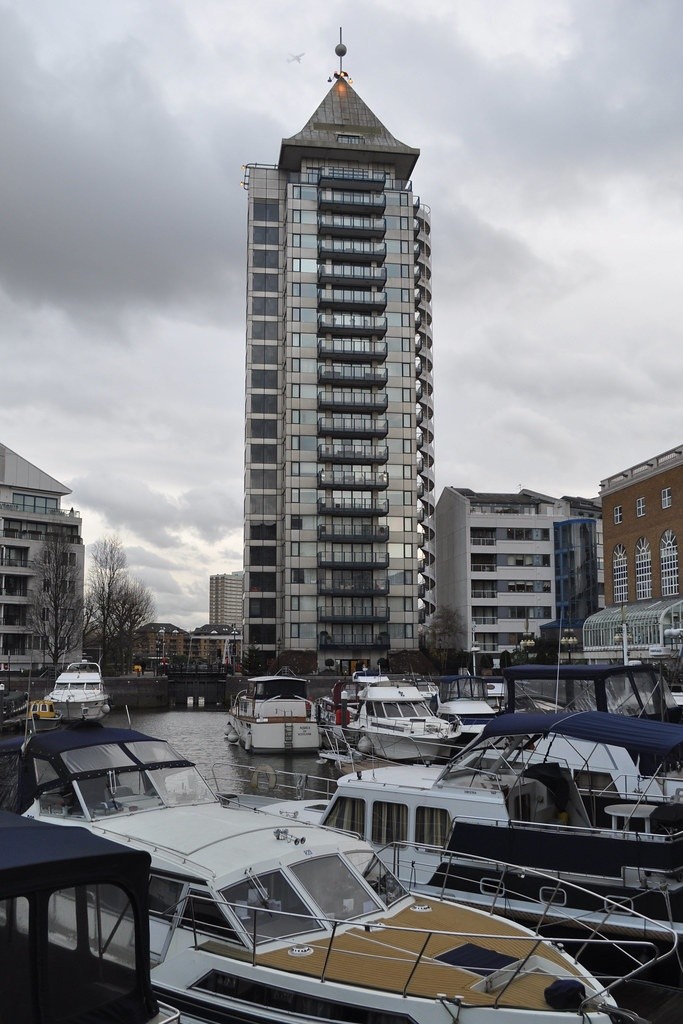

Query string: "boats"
[[204, 628, 683, 995], [22, 699, 61, 732], [0, 717, 647, 1024], [47, 659, 111, 725], [0, 650, 30, 734]]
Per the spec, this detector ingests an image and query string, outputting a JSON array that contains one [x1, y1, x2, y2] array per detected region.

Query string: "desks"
[[604, 804, 658, 832]]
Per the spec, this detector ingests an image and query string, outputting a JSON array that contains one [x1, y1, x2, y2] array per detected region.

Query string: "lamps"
[[327, 71, 337, 82], [340, 70, 353, 84]]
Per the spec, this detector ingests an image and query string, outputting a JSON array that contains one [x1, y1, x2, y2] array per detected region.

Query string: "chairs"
[[104, 786, 133, 802]]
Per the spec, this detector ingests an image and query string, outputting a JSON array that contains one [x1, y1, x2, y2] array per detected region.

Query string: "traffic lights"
[[232, 644, 237, 657]]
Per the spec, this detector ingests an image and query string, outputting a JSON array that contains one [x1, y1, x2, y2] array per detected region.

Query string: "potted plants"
[[320, 631, 331, 644], [377, 632, 389, 645]]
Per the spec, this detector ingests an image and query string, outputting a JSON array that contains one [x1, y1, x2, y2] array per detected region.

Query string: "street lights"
[[172, 629, 179, 656], [158, 627, 165, 673], [231, 629, 238, 676]]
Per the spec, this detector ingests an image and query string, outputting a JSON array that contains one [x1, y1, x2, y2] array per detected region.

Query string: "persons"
[[140, 661, 146, 675], [134, 662, 142, 677]]
